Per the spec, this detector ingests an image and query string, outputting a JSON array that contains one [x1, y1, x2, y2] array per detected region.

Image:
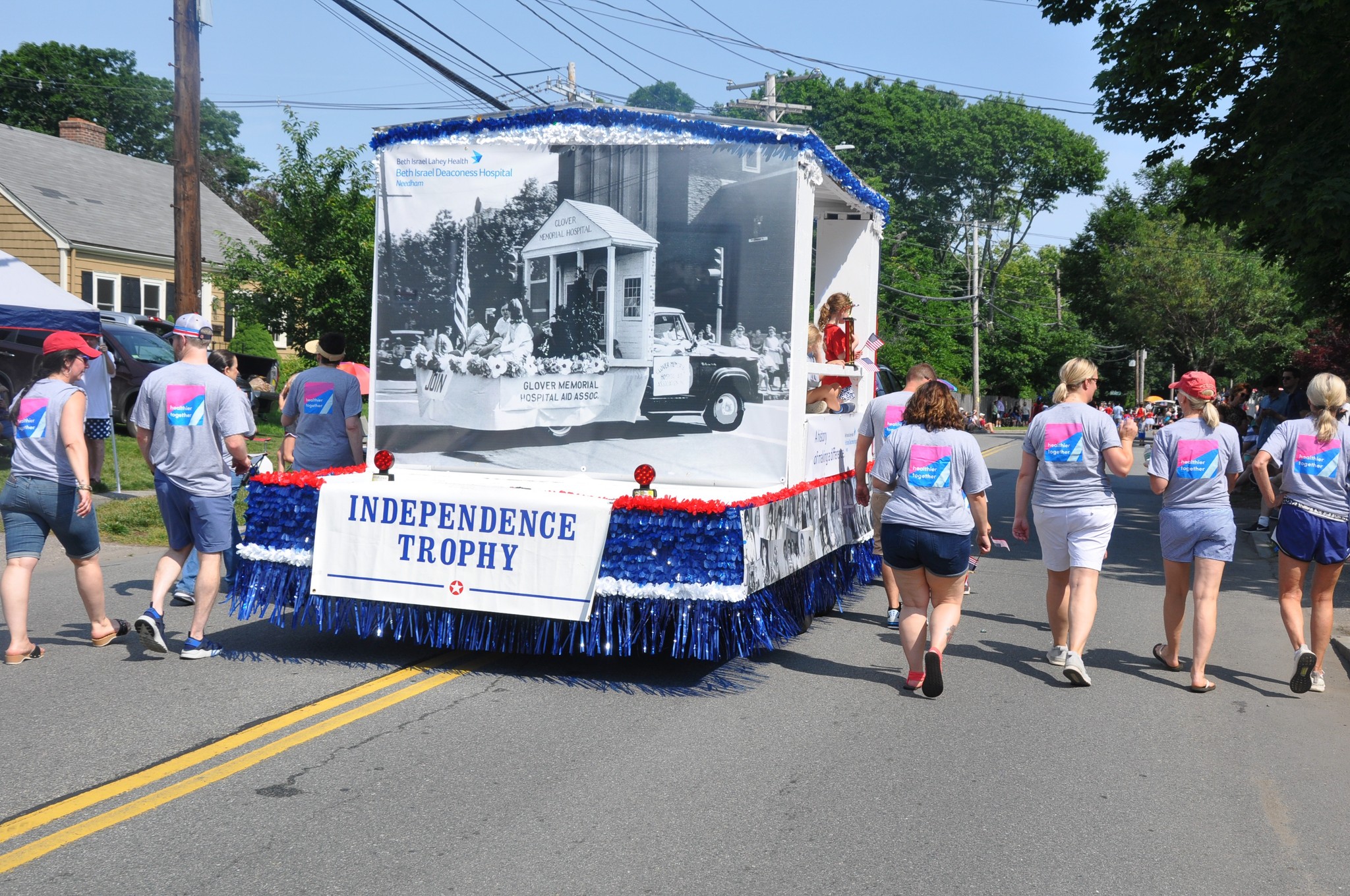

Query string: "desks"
[[1002, 417, 1010, 427]]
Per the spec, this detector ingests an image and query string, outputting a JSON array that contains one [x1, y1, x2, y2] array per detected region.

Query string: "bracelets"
[[76, 484, 92, 492]]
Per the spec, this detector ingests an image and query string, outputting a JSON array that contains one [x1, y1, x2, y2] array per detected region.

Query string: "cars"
[[875, 362, 904, 398], [0, 307, 216, 442]]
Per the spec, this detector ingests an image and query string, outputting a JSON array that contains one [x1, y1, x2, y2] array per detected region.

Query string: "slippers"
[[5, 643, 44, 664], [1152, 643, 1183, 671], [91, 619, 132, 648], [1191, 679, 1216, 692]]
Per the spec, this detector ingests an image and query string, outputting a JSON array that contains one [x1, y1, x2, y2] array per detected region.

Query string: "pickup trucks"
[[410, 305, 763, 444]]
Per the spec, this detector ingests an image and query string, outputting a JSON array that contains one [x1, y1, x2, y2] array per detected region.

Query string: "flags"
[[865, 333, 884, 352], [988, 533, 1011, 553], [854, 357, 880, 373], [969, 555, 979, 573]]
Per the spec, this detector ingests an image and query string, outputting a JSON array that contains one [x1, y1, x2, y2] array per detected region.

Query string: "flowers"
[[410, 344, 609, 379]]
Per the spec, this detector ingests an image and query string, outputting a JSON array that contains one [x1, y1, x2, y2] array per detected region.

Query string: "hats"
[[42, 331, 102, 360], [959, 407, 985, 416], [1167, 371, 1216, 401], [305, 339, 346, 361], [163, 312, 214, 340]]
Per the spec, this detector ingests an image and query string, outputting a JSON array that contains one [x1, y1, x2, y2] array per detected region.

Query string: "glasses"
[[1281, 376, 1293, 381], [1089, 376, 1101, 387], [1174, 388, 1179, 395], [1240, 392, 1250, 398], [76, 354, 89, 364]]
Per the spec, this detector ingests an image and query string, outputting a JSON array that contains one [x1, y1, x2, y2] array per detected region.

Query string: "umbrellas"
[[335, 361, 369, 394], [936, 378, 957, 392], [1145, 396, 1163, 403]]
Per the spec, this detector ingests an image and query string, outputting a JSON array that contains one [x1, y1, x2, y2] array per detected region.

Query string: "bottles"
[[1143, 445, 1151, 465]]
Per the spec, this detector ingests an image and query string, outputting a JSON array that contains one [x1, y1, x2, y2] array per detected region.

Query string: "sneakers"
[[1289, 644, 1317, 694], [1063, 652, 1092, 686], [173, 592, 196, 605], [134, 607, 169, 653], [886, 608, 901, 629], [1249, 472, 1258, 485], [1047, 640, 1068, 666], [1241, 521, 1271, 534], [1310, 669, 1326, 692], [180, 631, 223, 659]]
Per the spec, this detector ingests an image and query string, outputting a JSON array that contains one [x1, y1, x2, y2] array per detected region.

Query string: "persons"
[[870, 380, 992, 697], [278, 329, 365, 476], [392, 297, 535, 375], [1146, 370, 1245, 692], [1252, 372, 1350, 695], [1092, 366, 1350, 534], [655, 313, 792, 396], [77, 332, 116, 493], [855, 363, 938, 629], [806, 324, 855, 414], [0, 384, 17, 458], [819, 292, 860, 400], [0, 331, 131, 665], [130, 310, 250, 659], [1011, 357, 1139, 686], [172, 349, 257, 602], [957, 397, 1049, 435]]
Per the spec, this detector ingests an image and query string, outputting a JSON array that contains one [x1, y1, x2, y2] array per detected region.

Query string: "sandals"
[[903, 671, 926, 690], [922, 648, 944, 697]]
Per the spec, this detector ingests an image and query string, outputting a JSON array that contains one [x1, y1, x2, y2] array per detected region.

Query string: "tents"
[[0, 248, 123, 494]]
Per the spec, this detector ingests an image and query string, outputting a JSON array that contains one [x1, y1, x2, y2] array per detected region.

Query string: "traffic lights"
[[705, 247, 724, 280]]
